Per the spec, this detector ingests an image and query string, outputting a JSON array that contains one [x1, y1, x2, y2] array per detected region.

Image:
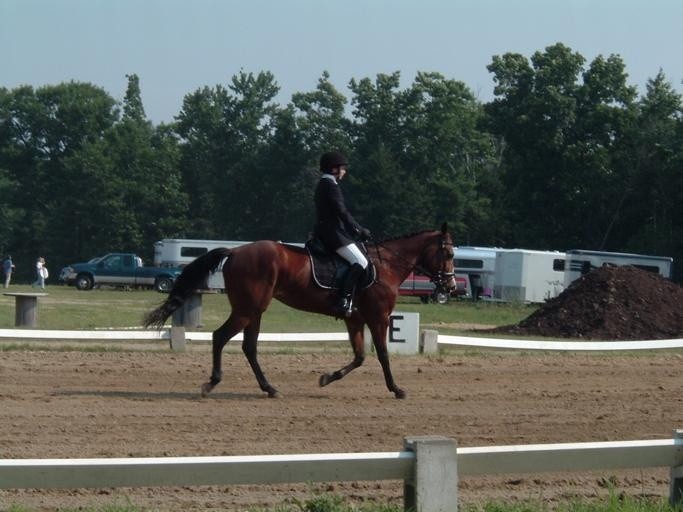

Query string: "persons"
[[29, 256, 45, 290], [311, 150, 372, 316], [1, 255, 16, 290]]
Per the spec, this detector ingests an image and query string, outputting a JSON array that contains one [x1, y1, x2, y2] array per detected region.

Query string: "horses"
[[138, 222, 459, 400]]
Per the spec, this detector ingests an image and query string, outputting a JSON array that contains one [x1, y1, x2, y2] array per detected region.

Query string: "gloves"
[[361, 228, 373, 240]]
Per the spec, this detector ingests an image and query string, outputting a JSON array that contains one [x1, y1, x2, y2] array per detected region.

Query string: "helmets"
[[320, 152, 349, 171]]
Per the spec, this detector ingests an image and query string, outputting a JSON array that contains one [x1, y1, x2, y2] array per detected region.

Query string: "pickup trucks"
[[59, 252, 185, 294], [397, 271, 468, 305]]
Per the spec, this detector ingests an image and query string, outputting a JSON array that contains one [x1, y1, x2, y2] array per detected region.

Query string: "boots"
[[328, 262, 358, 313]]
